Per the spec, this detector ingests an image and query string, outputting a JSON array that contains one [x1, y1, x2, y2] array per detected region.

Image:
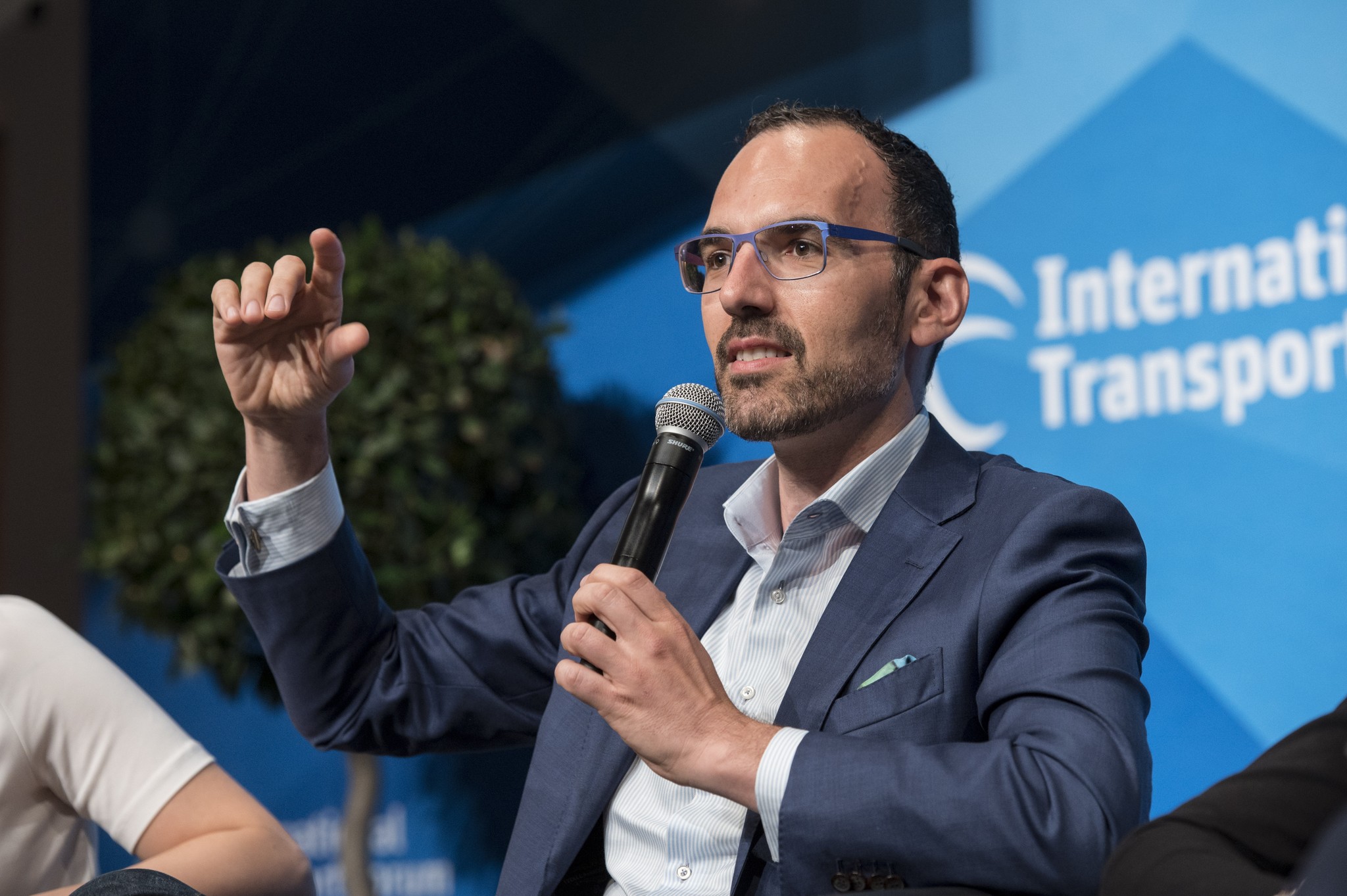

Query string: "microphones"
[[579, 384, 726, 675]]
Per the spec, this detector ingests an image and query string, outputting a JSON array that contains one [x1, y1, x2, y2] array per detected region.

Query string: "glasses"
[[675, 220, 938, 295]]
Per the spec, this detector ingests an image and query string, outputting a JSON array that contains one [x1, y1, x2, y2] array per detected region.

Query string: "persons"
[[0, 595, 312, 896], [212, 98, 1153, 896], [1100, 698, 1347, 896]]
